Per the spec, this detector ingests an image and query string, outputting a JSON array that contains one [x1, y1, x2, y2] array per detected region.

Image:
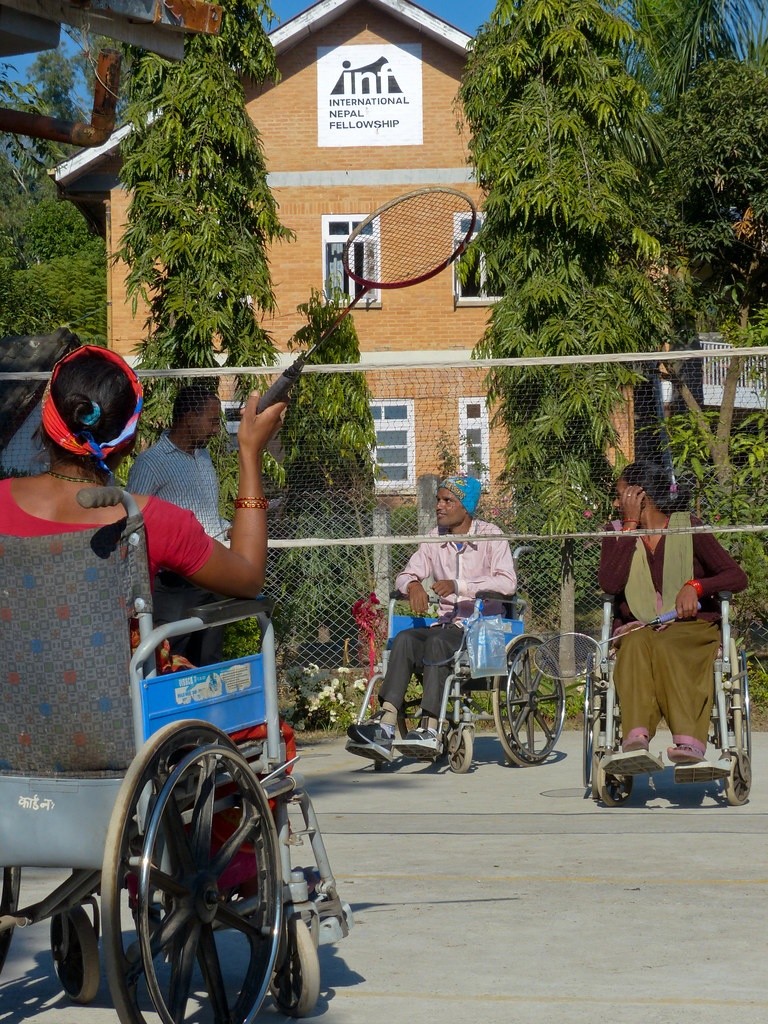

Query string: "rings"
[[627, 493, 631, 496]]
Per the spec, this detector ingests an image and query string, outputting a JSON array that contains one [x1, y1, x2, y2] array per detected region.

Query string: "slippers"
[[667, 745, 707, 763], [622, 737, 649, 753]]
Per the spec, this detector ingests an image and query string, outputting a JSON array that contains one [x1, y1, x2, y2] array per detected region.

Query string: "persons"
[[1, 344, 296, 914], [347, 476, 518, 757], [126, 385, 233, 667], [598, 460, 749, 763]]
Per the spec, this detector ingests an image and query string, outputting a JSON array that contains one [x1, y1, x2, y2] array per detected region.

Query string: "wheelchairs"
[[345, 546, 566, 775], [0, 486, 354, 1024], [582, 590, 753, 807]]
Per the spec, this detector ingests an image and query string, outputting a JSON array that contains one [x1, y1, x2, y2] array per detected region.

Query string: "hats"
[[435, 476, 481, 515]]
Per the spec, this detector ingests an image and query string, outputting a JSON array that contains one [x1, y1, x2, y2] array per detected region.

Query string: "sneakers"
[[347, 723, 395, 757], [407, 727, 436, 740]]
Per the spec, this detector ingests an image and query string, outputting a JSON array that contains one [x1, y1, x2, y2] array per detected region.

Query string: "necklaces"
[[46, 470, 102, 485]]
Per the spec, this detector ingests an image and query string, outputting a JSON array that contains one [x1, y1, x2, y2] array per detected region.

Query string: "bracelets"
[[235, 496, 269, 509], [685, 579, 703, 597], [621, 518, 639, 532]]
